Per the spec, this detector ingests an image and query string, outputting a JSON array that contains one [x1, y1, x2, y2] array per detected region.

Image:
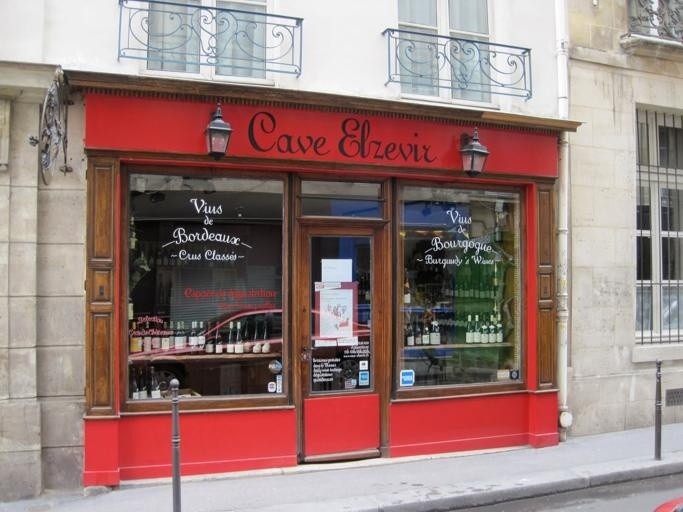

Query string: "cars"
[[126, 307, 371, 397]]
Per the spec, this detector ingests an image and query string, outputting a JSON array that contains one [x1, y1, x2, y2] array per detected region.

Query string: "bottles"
[[405, 311, 442, 346], [465, 312, 504, 344], [128, 318, 271, 354], [129, 366, 161, 399]]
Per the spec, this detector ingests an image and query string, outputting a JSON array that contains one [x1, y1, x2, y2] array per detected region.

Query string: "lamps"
[[203, 101, 234, 162], [457, 125, 492, 179]]
